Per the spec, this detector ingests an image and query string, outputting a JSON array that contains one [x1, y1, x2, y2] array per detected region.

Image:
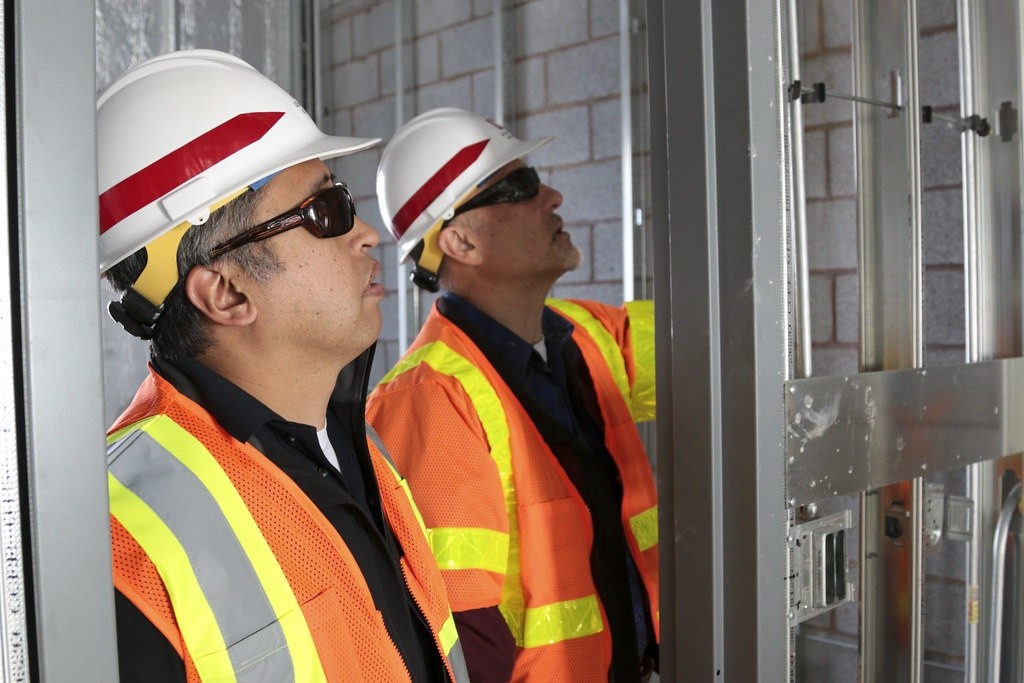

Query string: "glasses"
[[208, 182, 356, 260], [440, 165, 541, 230]]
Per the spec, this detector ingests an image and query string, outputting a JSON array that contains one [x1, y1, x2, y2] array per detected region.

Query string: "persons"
[[365, 107, 661, 682], [95, 47, 470, 682]]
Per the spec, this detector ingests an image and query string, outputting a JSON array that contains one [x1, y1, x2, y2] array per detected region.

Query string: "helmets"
[[96, 50, 382, 279], [376, 107, 555, 265]]
